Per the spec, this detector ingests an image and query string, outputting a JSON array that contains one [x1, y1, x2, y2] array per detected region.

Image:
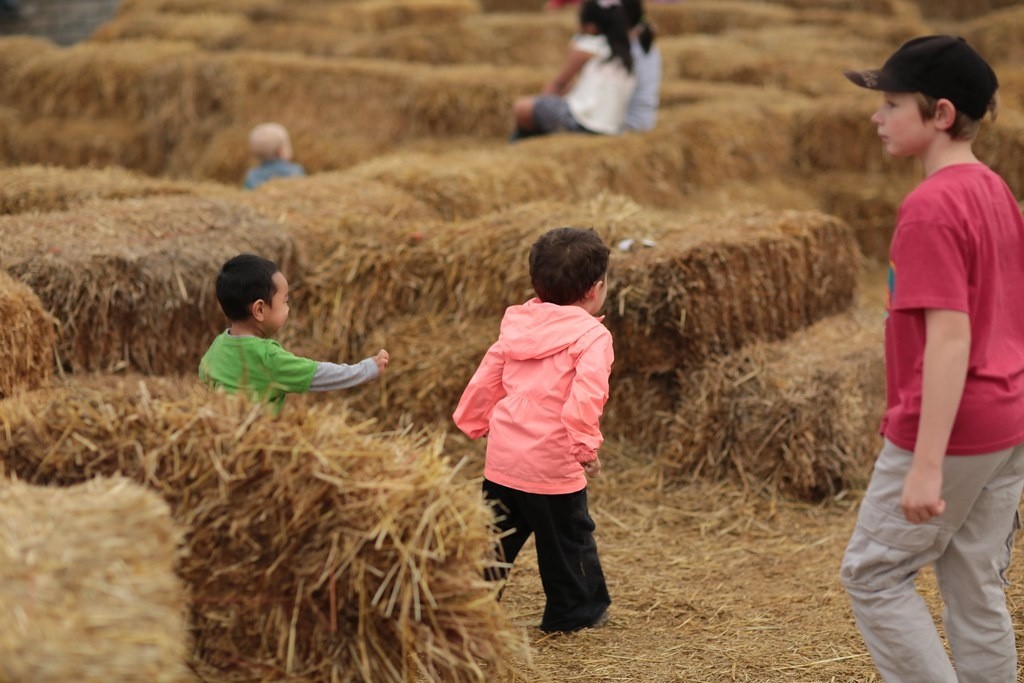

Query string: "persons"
[[452, 227, 614, 633], [512, 0, 636, 135], [547, 0, 661, 133], [844, 35, 1024, 683], [244, 121, 303, 189], [198, 255, 389, 410]]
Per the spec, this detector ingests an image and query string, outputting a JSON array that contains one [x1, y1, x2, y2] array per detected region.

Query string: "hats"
[[842, 35, 1000, 121]]
